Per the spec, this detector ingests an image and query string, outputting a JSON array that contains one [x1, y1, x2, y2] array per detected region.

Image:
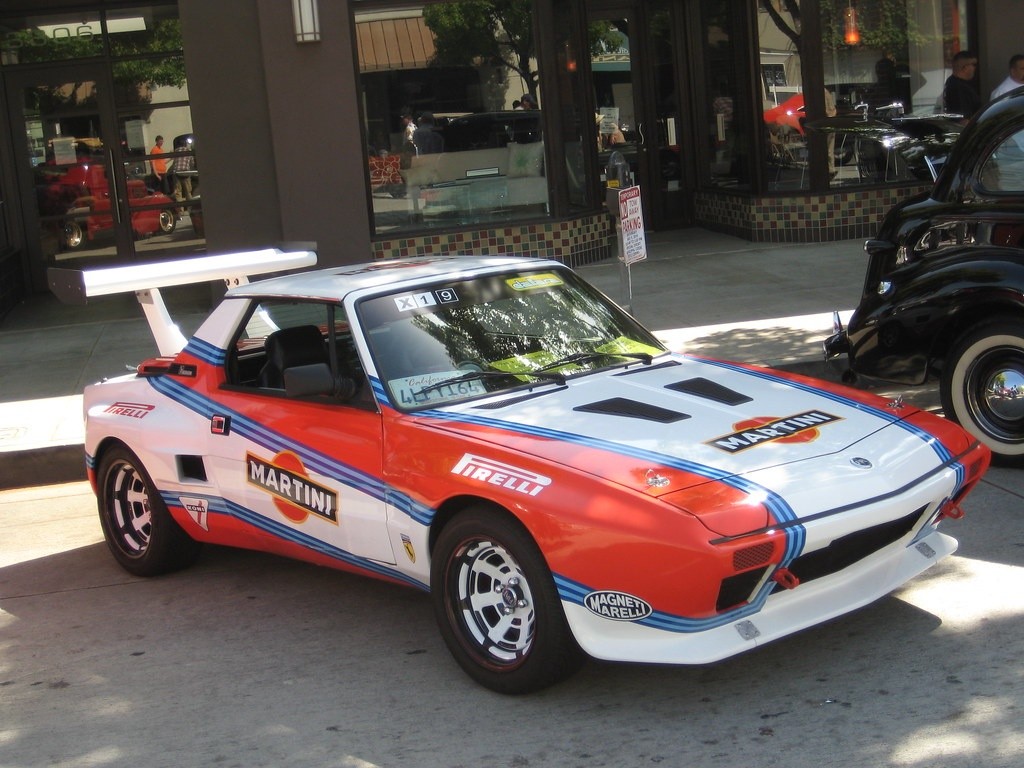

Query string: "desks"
[[801, 117, 898, 189]]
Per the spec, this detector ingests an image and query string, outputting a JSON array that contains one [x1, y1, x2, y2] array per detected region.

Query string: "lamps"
[[843, 0, 860, 45]]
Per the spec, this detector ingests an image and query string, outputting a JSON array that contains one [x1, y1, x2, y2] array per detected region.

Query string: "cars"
[[35, 133, 196, 252], [84, 253, 992, 702], [823, 88, 1024, 468], [765, 94, 807, 137]]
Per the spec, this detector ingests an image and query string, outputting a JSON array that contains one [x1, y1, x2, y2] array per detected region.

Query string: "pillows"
[[508, 142, 544, 177]]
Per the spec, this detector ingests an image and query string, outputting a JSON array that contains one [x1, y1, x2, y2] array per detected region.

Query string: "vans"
[[389, 109, 544, 201]]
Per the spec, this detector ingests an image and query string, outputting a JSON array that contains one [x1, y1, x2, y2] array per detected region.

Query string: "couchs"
[[405, 142, 549, 219]]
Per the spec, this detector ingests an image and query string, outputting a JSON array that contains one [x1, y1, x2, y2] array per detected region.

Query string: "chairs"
[[263, 325, 335, 399], [768, 120, 809, 186]]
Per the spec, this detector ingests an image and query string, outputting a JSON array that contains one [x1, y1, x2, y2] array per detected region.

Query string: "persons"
[[173, 140, 192, 220], [413, 112, 444, 156], [990, 54, 1024, 148], [944, 51, 979, 125], [506, 94, 542, 144], [400, 113, 418, 156], [825, 88, 838, 181], [864, 57, 927, 115], [149, 135, 172, 196]]
